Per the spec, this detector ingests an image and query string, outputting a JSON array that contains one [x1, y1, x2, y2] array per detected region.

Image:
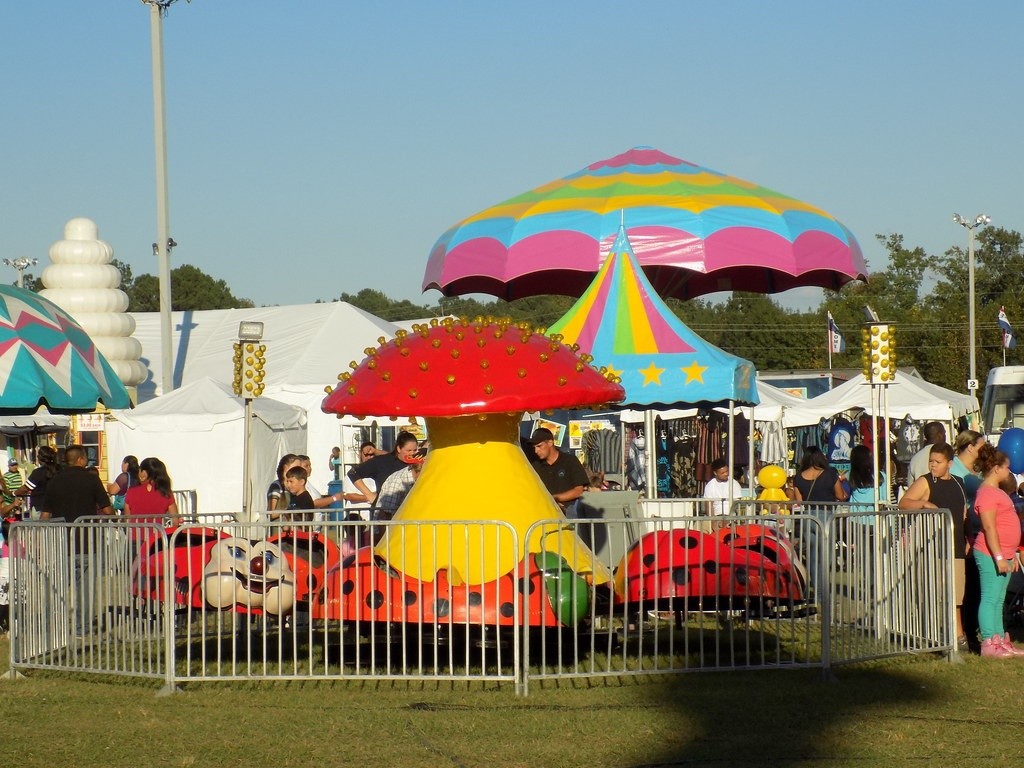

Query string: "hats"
[[404, 448, 427, 464], [8, 458, 18, 466], [527, 428, 554, 445]]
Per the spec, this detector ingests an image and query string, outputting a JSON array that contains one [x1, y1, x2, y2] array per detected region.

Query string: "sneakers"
[[981, 634, 1015, 657], [1001, 632, 1024, 656]]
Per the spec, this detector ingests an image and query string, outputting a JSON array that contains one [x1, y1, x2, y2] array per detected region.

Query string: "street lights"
[[953, 211, 992, 399]]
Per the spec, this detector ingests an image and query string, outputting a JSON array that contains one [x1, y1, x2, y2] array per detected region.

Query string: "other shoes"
[[959, 635, 974, 649]]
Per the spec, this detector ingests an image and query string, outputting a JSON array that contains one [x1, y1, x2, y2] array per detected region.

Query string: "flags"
[[824, 305, 1015, 349]]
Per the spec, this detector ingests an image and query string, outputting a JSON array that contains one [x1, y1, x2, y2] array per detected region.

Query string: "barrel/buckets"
[[328, 480, 343, 521]]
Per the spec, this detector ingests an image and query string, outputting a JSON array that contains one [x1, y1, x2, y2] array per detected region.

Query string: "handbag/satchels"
[[113, 472, 130, 510], [794, 519, 800, 538]]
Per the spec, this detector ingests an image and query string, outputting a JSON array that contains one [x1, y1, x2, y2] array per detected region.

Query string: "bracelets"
[[994, 555, 1003, 561], [332, 494, 337, 501]]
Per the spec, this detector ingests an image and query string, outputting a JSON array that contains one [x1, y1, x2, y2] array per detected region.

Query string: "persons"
[[528, 421, 1024, 661], [0, 441, 181, 641]]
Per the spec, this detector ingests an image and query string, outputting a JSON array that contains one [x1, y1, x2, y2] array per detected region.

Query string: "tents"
[[108, 300, 466, 540], [534, 225, 982, 512]]
